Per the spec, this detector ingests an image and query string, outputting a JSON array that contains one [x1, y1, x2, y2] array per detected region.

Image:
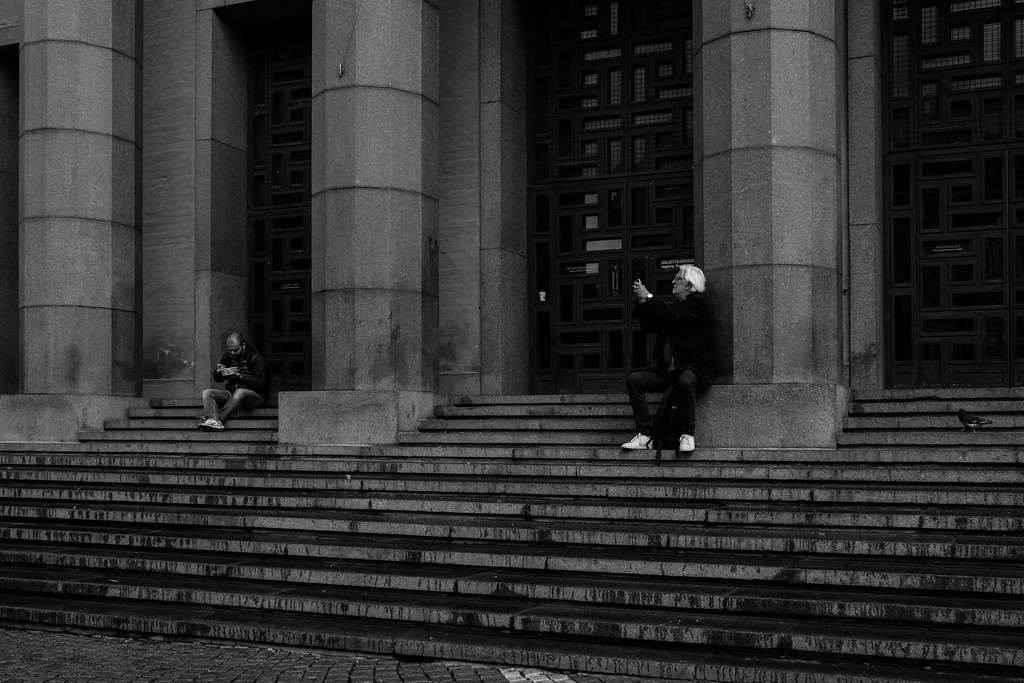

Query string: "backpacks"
[[645, 381, 686, 466]]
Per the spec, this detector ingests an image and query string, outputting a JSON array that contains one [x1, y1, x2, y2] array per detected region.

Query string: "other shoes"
[[197, 418, 226, 432]]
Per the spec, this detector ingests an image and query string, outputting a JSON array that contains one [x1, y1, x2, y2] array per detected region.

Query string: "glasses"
[[674, 276, 686, 282]]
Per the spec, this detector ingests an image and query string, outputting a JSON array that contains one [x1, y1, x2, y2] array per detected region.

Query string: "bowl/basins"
[[220, 367, 239, 376]]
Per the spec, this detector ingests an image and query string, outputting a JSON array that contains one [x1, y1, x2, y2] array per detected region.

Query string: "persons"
[[621, 264, 716, 451], [197, 332, 269, 430]]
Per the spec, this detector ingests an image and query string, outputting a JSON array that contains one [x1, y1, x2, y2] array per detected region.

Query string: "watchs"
[[646, 294, 654, 300]]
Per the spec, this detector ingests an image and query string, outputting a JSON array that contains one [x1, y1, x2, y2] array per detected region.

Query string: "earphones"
[[243, 345, 245, 349]]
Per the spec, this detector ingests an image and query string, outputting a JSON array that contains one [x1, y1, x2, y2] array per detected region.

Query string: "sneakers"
[[678, 434, 695, 452], [621, 431, 654, 450]]
